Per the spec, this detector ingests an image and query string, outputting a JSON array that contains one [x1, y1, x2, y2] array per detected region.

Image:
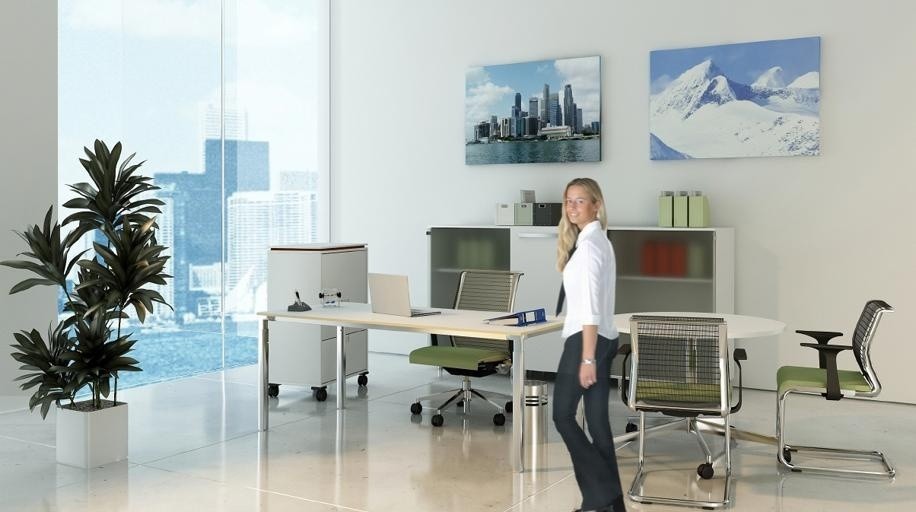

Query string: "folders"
[[482, 309, 545, 326]]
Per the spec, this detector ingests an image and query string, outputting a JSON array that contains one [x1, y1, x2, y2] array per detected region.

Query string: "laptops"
[[367, 273, 441, 317]]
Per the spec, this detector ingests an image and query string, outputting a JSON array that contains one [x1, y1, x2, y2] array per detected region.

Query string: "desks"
[[613, 312, 791, 479], [257, 299, 566, 475]]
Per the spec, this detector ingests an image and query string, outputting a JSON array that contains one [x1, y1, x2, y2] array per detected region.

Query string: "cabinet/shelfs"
[[268, 240, 370, 403]]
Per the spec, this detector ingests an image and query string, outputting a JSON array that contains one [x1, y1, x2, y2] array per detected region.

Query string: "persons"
[[552, 177, 626, 512]]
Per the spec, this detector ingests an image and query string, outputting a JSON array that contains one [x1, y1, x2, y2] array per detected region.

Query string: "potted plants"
[[0, 135, 179, 472]]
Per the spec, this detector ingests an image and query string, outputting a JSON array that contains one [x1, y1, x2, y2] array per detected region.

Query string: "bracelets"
[[581, 358, 596, 366]]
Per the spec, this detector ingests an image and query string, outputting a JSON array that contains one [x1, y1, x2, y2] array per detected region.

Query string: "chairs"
[[612, 312, 748, 510], [774, 298, 896, 480], [407, 271, 524, 428]]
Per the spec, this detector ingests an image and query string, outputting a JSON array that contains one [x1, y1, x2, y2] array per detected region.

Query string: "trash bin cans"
[[523, 380, 548, 444]]
